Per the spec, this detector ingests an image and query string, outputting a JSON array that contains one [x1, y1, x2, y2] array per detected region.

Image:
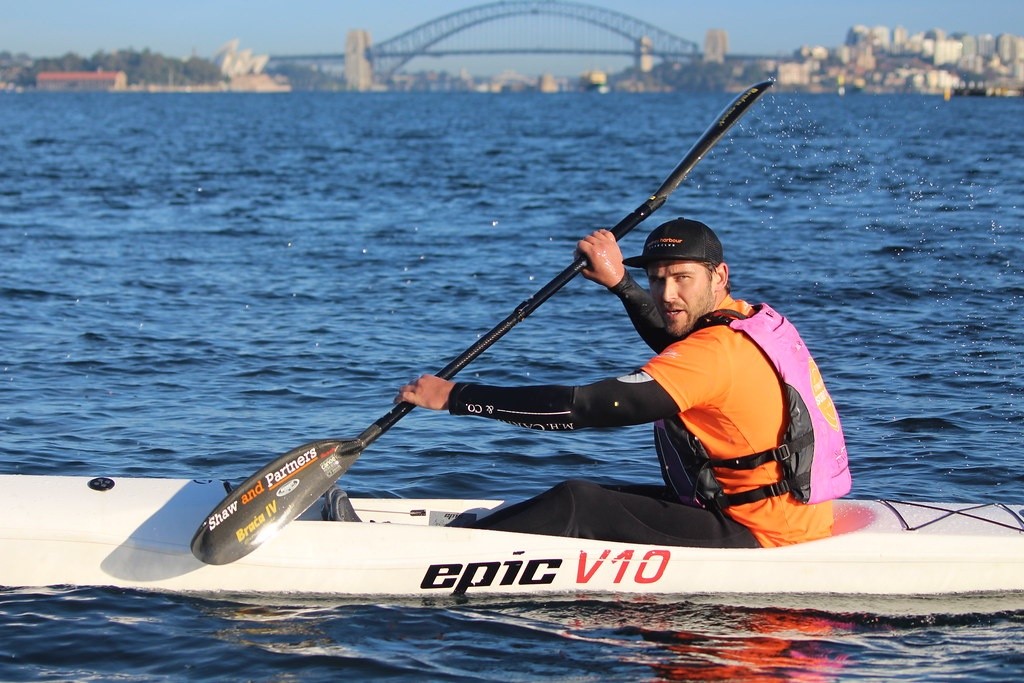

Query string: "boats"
[[1, 474, 1024, 618]]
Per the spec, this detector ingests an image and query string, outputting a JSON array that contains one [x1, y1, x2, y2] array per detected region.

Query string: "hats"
[[621, 216, 723, 267]]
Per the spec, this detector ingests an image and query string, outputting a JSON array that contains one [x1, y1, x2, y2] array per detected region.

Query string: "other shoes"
[[324, 486, 361, 522]]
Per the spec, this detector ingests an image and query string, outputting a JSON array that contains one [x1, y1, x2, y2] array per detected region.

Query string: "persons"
[[320, 217, 852, 549]]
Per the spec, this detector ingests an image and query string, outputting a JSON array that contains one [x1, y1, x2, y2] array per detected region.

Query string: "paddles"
[[189, 81, 775, 566]]
